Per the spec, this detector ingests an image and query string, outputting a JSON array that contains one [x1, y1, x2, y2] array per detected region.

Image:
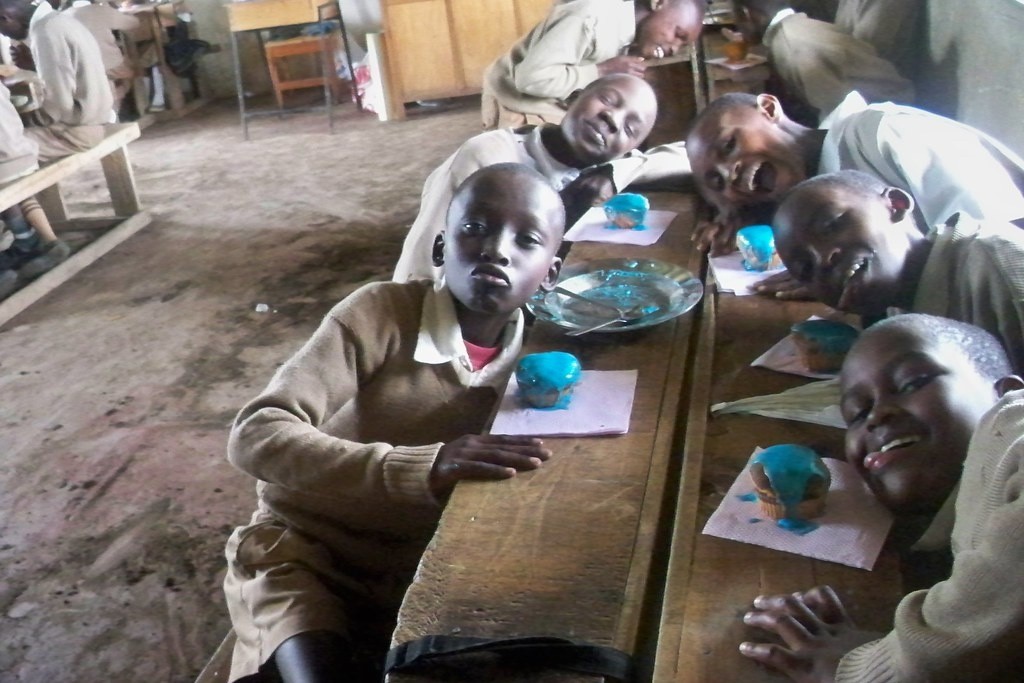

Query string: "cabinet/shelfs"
[[377, 0, 561, 126]]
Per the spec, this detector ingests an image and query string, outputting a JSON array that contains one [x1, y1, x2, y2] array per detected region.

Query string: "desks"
[[640, 28, 770, 115], [220, 0, 363, 140], [386, 178, 908, 680], [115, 1, 210, 130]]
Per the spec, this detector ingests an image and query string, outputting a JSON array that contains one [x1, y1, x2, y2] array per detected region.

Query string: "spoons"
[[552, 286, 645, 320]]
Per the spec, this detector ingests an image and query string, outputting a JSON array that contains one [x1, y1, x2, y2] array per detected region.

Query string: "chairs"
[[265, 31, 343, 112]]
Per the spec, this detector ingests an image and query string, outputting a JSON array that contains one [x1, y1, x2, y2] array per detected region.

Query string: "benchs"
[[0, 122, 151, 331]]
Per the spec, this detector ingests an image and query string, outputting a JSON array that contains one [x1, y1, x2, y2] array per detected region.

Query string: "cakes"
[[514, 351, 580, 407], [792, 320, 857, 373], [723, 41, 748, 60], [736, 225, 776, 265], [603, 193, 648, 227], [749, 445, 830, 520]]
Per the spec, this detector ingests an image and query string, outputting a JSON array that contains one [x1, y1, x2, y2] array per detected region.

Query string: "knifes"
[[566, 305, 660, 335]]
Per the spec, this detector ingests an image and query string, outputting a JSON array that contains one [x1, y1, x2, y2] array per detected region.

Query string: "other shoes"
[[0, 230, 41, 271], [149, 104, 165, 112], [24, 241, 71, 280]]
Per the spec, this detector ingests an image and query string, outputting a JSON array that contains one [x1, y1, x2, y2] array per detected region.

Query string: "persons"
[[390, 72, 694, 283], [0, 80, 71, 304], [0, 0, 184, 114], [773, 167, 1024, 378], [736, 313, 1024, 683], [0, 0, 119, 254], [686, 86, 1024, 302], [834, 0, 913, 74], [481, 1, 708, 132], [221, 161, 567, 683], [730, 0, 916, 129]]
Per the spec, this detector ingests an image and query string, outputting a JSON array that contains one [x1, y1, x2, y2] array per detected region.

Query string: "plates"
[[525, 258, 703, 332]]
